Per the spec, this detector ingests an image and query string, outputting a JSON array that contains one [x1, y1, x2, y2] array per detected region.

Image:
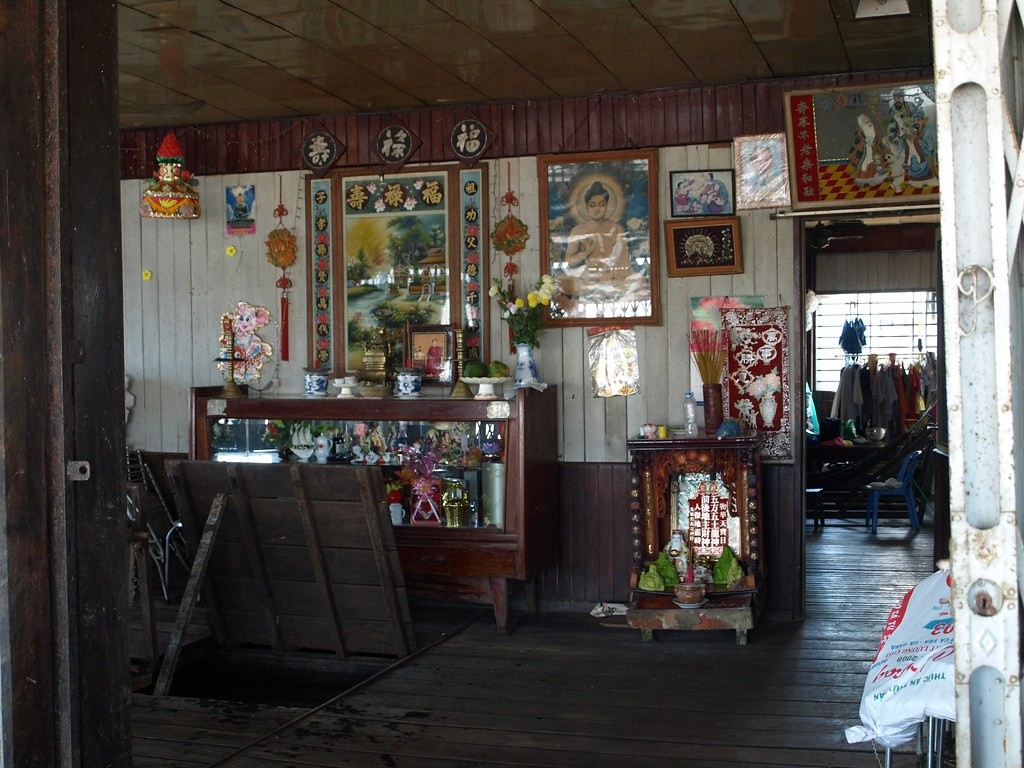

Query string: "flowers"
[[488, 272, 559, 349]]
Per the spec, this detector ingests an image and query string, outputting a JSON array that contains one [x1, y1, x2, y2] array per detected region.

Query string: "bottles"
[[682, 392, 698, 437]]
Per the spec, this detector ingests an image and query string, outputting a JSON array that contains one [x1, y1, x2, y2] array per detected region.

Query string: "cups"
[[397, 373, 421, 397], [389, 503, 406, 526], [305, 374, 329, 395]]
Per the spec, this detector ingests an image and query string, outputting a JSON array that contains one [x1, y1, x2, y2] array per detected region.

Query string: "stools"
[[805, 488, 825, 531]]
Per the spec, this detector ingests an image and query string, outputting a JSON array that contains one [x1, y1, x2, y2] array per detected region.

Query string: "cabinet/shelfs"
[[184, 378, 562, 633]]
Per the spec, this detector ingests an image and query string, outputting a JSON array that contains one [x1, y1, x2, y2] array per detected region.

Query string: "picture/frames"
[[663, 219, 744, 279], [734, 135, 792, 212], [402, 321, 458, 387], [668, 168, 735, 217], [535, 147, 663, 326]]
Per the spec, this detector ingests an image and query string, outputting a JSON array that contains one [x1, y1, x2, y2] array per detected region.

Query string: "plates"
[[672, 430, 685, 434], [672, 598, 708, 608]]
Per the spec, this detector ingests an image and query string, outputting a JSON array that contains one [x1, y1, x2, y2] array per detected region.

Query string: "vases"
[[512, 343, 538, 388]]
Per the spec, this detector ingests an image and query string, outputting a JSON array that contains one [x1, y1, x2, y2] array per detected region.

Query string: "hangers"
[[843, 353, 923, 369]]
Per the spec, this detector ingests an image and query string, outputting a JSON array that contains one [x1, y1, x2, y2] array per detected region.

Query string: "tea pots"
[[314, 432, 333, 464]]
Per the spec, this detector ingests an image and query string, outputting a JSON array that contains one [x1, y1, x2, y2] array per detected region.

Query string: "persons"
[[664, 530, 690, 581], [427, 338, 441, 374]]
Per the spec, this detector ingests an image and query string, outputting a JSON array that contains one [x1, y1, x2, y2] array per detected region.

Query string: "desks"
[[818, 443, 887, 486]]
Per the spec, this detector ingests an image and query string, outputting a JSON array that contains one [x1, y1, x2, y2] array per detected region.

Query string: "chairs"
[[866, 450, 924, 534]]
